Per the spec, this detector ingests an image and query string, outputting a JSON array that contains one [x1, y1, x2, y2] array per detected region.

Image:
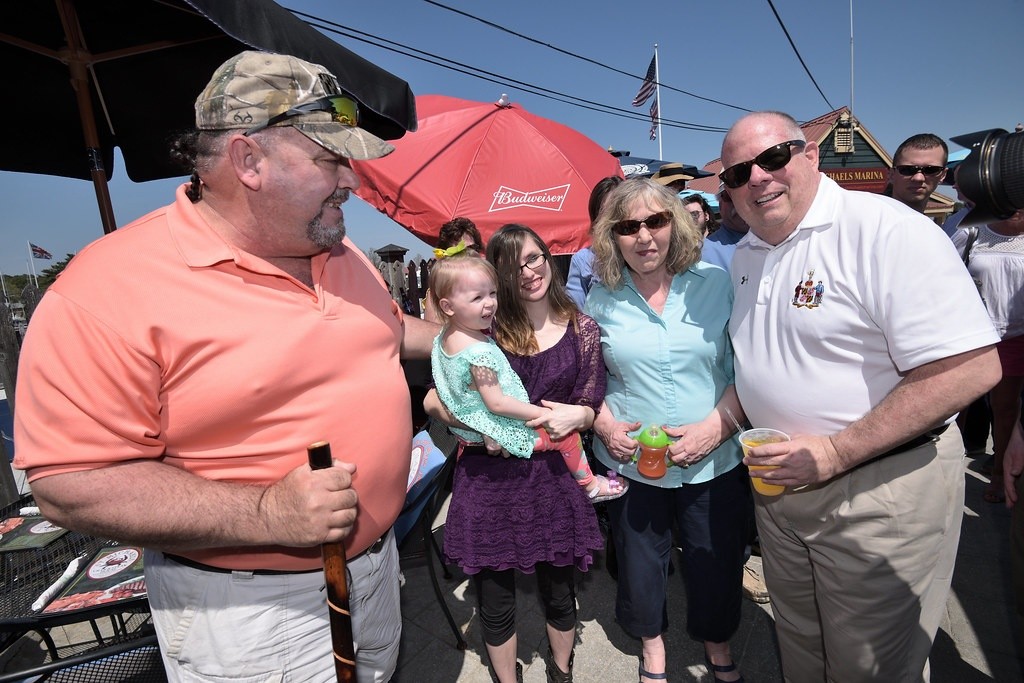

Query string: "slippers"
[[704, 651, 745, 683], [639, 656, 668, 683]]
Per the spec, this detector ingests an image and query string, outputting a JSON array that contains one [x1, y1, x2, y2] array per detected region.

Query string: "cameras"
[[949, 128, 1024, 229]]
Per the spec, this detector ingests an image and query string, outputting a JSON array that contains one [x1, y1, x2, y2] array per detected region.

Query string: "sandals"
[[587, 470, 630, 503]]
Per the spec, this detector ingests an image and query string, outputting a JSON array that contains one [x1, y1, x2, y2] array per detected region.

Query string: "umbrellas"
[[349, 93, 626, 257], [0, 0, 417, 236], [608, 145, 715, 178]]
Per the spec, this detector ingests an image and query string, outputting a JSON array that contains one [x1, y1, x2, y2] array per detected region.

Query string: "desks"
[[0, 488, 152, 664]]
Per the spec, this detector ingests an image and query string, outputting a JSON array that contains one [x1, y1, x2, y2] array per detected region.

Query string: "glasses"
[[894, 165, 945, 176], [690, 210, 704, 218], [673, 180, 685, 185], [718, 140, 806, 189], [503, 254, 547, 282], [720, 190, 732, 202], [244, 94, 360, 137], [611, 211, 674, 236]]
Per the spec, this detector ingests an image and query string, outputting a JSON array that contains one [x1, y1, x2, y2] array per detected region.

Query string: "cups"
[[739, 428, 791, 496]]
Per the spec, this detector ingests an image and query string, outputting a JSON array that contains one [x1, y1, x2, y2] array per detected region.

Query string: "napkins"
[[19, 506, 40, 516], [32, 556, 87, 612]]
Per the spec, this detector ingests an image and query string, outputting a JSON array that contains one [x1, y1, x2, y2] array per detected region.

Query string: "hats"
[[194, 50, 395, 160], [650, 163, 694, 186]]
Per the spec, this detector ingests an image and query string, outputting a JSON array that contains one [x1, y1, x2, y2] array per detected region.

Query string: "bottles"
[[399, 287, 415, 315], [629, 423, 677, 477]]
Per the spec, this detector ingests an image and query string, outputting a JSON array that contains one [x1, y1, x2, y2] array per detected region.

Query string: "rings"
[[684, 464, 689, 468], [620, 454, 624, 460]]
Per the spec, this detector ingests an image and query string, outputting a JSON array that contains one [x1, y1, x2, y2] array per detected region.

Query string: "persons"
[[888, 133, 949, 214], [424, 219, 480, 325], [941, 162, 1024, 508], [720, 112, 1004, 681], [649, 164, 752, 268], [13, 51, 443, 683], [424, 224, 608, 683], [580, 177, 756, 682], [431, 242, 629, 502], [566, 175, 622, 312]]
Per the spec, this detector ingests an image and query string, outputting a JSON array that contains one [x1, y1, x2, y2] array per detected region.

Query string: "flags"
[[631, 54, 656, 107], [650, 97, 658, 140], [29, 242, 51, 259]]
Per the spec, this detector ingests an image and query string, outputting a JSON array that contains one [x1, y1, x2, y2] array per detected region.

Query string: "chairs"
[[393, 420, 466, 653], [0, 635, 163, 683]]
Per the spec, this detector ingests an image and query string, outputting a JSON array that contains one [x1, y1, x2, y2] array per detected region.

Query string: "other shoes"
[[492, 662, 523, 683], [983, 479, 1005, 502], [545, 649, 575, 683]]
